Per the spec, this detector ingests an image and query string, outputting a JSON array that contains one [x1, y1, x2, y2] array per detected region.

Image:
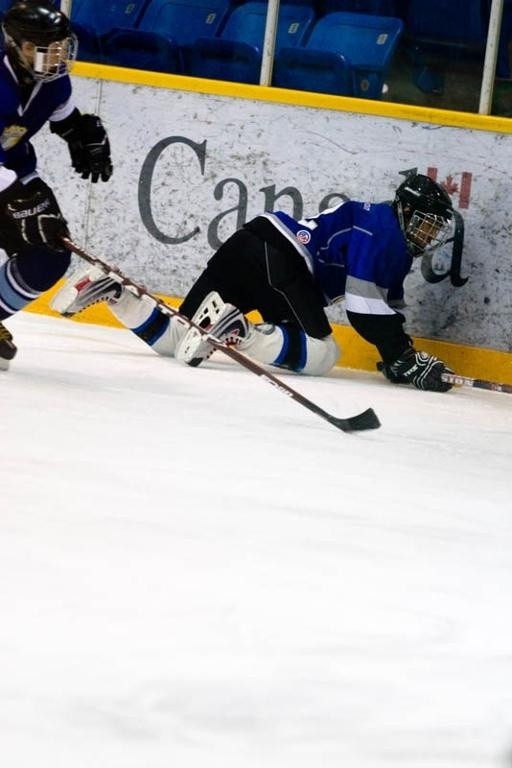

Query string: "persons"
[[51, 174, 457, 394], [2, 1, 115, 359]]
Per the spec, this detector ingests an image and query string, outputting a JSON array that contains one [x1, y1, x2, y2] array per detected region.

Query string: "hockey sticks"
[[63, 238, 381, 431]]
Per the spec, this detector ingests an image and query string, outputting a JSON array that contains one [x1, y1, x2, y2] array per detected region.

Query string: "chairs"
[[0, 0, 512, 100]]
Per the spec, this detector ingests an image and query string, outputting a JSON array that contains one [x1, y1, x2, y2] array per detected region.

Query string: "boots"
[[60, 272, 125, 317], [188, 304, 252, 366], [0, 324, 17, 359]]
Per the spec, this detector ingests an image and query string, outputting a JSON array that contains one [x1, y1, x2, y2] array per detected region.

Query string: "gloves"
[[374, 348, 455, 392], [6, 177, 71, 252], [69, 112, 112, 183]]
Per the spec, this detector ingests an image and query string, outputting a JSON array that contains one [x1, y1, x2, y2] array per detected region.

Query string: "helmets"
[[3, 2, 79, 83], [391, 173, 452, 258]]
